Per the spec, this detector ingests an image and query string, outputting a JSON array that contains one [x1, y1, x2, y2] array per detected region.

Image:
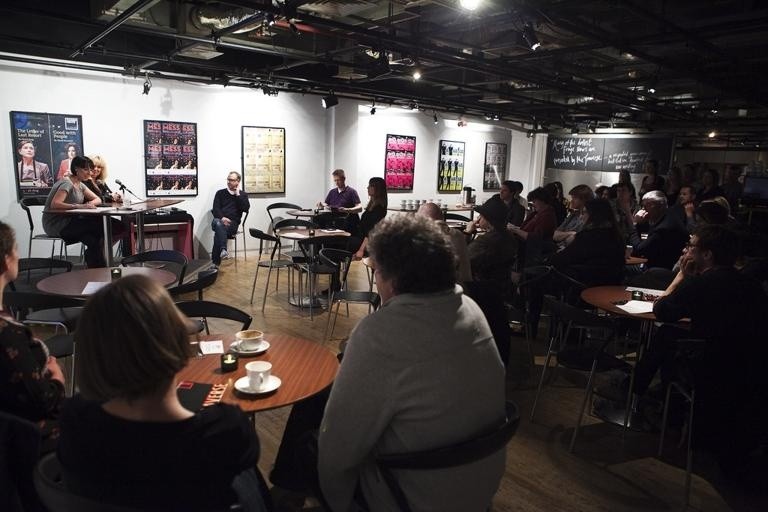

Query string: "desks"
[[173, 335, 341, 428]]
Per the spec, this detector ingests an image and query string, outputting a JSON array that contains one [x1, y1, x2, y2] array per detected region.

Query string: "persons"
[[56, 143, 82, 180], [39, 273, 261, 510], [321, 177, 388, 296], [81, 156, 128, 260], [41, 155, 125, 268], [263, 210, 506, 509], [305, 169, 362, 237], [471, 165, 768, 400], [0, 220, 66, 458], [210, 171, 250, 269], [16, 139, 53, 195]]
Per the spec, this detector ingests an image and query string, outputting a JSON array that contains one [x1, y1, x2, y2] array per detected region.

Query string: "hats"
[[693, 201, 729, 224], [473, 198, 508, 231]]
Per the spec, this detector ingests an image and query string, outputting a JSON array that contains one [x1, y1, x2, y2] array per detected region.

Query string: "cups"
[[235, 330, 264, 351], [401, 198, 448, 208], [244, 361, 273, 391]]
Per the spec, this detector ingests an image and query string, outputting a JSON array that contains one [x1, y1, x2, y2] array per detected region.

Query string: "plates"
[[229, 340, 271, 356], [233, 375, 282, 395]]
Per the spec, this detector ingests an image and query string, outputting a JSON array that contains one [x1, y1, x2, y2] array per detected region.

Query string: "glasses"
[[228, 179, 235, 182], [680, 241, 702, 249]]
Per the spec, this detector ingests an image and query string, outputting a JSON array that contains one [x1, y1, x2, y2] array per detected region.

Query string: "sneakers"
[[322, 283, 341, 294], [220, 251, 228, 259], [508, 320, 536, 337], [592, 376, 628, 403]]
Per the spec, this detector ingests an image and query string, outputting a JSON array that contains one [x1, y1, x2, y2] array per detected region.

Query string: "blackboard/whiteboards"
[[545, 135, 605, 170], [602, 137, 673, 175]]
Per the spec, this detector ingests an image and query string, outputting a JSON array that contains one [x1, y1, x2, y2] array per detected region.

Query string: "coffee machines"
[[462, 184, 477, 208]]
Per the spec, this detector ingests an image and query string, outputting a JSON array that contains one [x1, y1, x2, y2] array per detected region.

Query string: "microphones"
[[115, 178, 128, 191]]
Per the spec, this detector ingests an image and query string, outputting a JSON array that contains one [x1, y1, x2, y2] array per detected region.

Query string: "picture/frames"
[[483, 142, 507, 190], [437, 140, 465, 191], [384, 134, 416, 190], [433, 113, 438, 124], [241, 125, 285, 194], [143, 120, 198, 197], [10, 110, 84, 203]]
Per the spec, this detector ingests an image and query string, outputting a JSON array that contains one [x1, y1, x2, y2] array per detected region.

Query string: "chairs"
[[30, 452, 236, 512], [225, 210, 249, 273], [175, 300, 252, 335], [0, 410, 42, 511]]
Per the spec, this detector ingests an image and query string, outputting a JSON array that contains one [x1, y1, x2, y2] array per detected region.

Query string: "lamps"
[[521, 21, 541, 51], [320, 88, 338, 109]]
